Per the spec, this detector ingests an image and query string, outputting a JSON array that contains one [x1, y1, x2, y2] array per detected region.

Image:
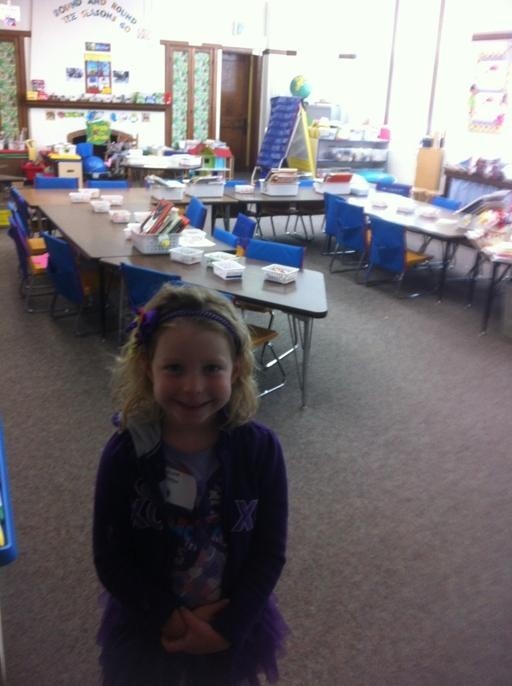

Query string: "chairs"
[[6, 161, 327, 416], [327, 173, 510, 304]]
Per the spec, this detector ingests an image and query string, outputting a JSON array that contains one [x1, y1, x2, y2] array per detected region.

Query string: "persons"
[[89, 279, 295, 685]]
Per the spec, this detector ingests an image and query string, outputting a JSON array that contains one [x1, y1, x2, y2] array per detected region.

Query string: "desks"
[[467, 231, 512, 335]]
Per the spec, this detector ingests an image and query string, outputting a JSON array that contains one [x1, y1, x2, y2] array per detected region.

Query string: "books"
[[322, 166, 354, 183], [262, 166, 301, 185], [140, 196, 191, 252]]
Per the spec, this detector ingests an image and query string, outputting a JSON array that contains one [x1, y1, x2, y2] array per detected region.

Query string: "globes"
[[290, 76, 311, 107]]
